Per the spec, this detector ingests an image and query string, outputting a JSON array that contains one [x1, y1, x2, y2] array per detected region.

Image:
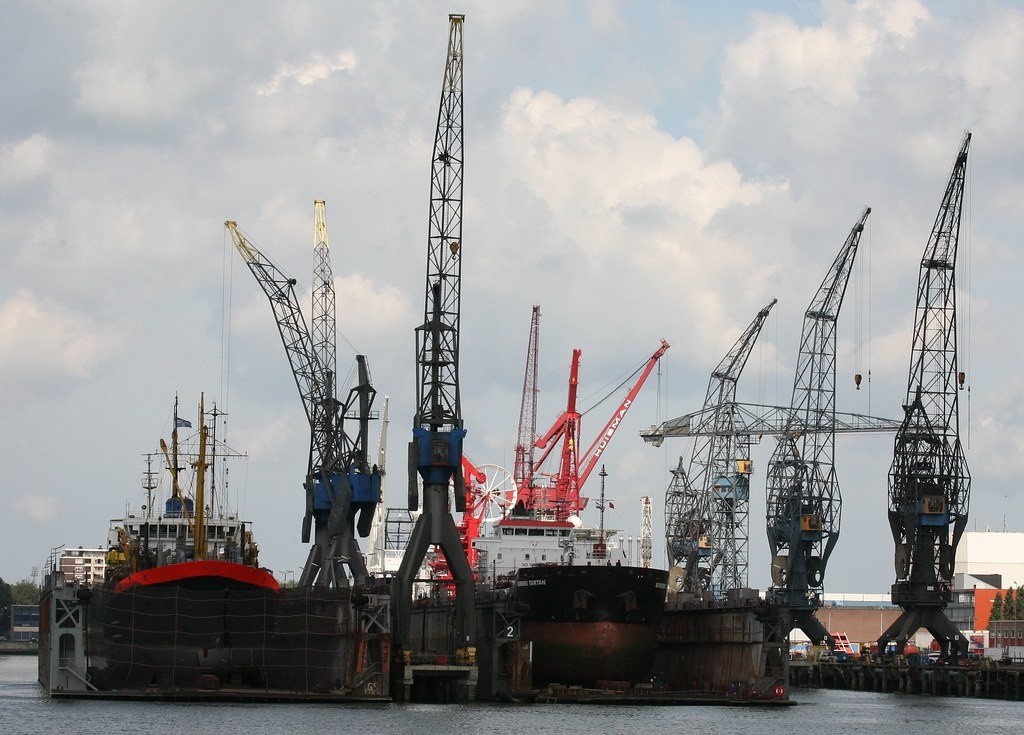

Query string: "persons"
[[607, 560, 612, 566], [616, 560, 621, 567]]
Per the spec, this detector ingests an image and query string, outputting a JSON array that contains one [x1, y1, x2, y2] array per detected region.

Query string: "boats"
[[101, 390, 281, 596], [470, 510, 625, 595]]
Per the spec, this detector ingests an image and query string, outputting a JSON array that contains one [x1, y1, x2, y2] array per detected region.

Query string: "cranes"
[[223, 197, 386, 586], [503, 303, 671, 521], [876, 131, 973, 654], [386, 12, 497, 707], [641, 207, 950, 649]]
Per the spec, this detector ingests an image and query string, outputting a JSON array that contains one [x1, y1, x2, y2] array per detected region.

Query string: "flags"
[[610, 502, 614, 509], [175, 416, 191, 428]]
